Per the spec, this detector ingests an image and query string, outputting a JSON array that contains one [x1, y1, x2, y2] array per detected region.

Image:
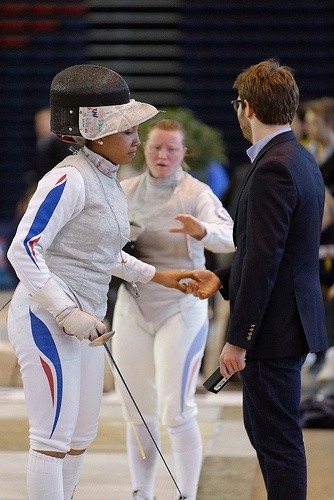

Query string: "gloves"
[[32, 277, 107, 342]]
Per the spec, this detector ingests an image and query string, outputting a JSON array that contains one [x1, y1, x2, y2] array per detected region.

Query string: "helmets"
[[50, 64, 131, 146]]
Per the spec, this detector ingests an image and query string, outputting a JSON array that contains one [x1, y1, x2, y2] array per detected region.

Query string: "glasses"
[[231, 99, 245, 112]]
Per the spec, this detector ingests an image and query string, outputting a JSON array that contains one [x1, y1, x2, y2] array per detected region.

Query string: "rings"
[[181, 269, 186, 272]]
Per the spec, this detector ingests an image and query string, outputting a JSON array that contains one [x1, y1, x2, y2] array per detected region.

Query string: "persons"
[[0, 64, 334, 500], [179, 61, 328, 499]]
